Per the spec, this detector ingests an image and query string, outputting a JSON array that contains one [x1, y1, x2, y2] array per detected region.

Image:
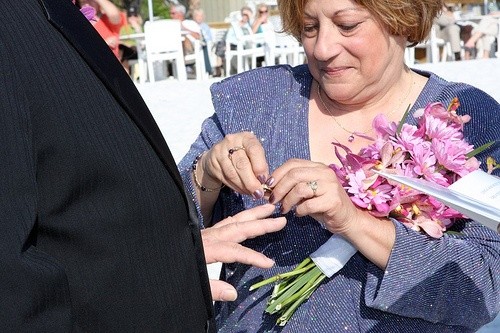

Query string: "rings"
[[307, 180, 319, 197], [227, 145, 246, 160], [260, 184, 274, 200]]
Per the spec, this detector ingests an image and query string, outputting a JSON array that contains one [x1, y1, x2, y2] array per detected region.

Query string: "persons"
[[174, 0, 500, 333], [0, 0, 287, 333], [72, 0, 145, 75], [168, 0, 221, 77], [433, 0, 500, 61], [231, 3, 279, 69]]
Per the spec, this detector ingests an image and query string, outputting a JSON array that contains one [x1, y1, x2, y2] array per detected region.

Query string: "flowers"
[[250, 95, 500, 328]]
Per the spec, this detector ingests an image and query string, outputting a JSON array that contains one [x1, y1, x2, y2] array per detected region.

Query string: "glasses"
[[169, 11, 177, 15], [259, 10, 268, 13]]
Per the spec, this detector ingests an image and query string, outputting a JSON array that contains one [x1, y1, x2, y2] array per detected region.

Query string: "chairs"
[[142, 14, 442, 84]]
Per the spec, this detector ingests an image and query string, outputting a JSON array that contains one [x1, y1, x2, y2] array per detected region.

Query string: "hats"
[[127, 4, 139, 17]]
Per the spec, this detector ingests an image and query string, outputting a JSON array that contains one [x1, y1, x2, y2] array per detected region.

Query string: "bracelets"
[[192, 150, 226, 192]]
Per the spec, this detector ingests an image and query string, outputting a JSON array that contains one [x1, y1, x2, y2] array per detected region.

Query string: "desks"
[[121, 28, 149, 81]]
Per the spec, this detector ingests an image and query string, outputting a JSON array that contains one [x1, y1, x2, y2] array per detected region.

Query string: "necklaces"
[[317, 61, 413, 142]]
